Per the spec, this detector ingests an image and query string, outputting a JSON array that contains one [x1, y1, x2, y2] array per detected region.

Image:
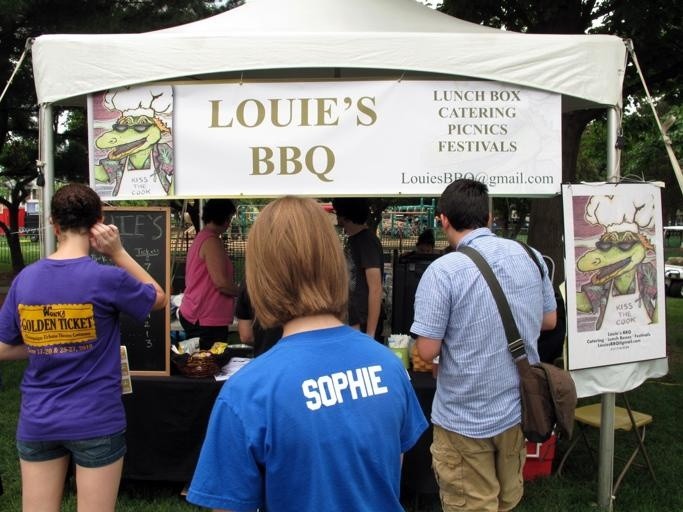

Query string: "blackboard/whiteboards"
[[83, 207, 171, 379]]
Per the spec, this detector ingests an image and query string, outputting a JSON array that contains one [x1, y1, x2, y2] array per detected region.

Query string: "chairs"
[[550, 353, 659, 512]]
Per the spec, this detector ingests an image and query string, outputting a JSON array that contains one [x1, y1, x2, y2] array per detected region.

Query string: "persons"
[[177, 198, 243, 367], [330, 198, 384, 342], [185, 197, 431, 512], [0, 181, 167, 511], [399, 226, 447, 257], [232, 278, 285, 357], [407, 177, 556, 512]]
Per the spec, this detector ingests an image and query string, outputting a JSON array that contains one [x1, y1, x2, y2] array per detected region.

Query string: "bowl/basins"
[[224, 343, 252, 360]]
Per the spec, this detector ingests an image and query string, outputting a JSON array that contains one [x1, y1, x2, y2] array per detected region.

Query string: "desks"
[[110, 357, 438, 483]]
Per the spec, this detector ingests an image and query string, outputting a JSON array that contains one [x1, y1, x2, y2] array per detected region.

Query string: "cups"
[[388, 347, 410, 369]]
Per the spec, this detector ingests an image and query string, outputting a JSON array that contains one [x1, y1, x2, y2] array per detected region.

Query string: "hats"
[[583, 194, 655, 233]]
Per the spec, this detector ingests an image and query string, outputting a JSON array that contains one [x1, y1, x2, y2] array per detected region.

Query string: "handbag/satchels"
[[518, 363, 579, 444]]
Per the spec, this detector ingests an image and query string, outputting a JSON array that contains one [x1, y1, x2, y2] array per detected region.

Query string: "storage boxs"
[[520, 429, 561, 481]]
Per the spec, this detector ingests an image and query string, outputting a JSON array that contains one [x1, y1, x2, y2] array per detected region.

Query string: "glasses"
[[595, 239, 638, 251]]
